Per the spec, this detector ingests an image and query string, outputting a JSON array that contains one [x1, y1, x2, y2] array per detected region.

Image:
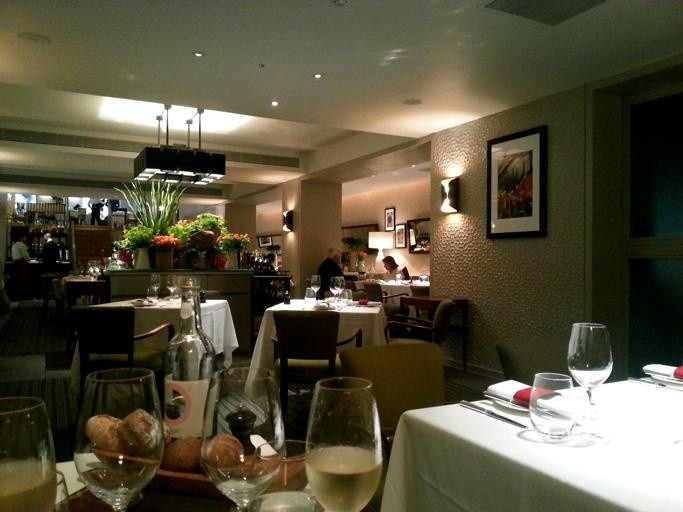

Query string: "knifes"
[[460, 399, 530, 428], [627, 374, 668, 388]]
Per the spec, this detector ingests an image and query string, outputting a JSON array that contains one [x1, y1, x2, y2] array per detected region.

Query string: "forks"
[[481, 390, 554, 417], [643, 366, 672, 379]]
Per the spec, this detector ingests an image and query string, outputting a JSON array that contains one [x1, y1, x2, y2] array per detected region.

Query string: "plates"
[[493, 398, 529, 413], [650, 375, 683, 387]]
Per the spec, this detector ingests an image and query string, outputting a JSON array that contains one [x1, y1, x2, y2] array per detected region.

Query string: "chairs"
[[39, 276, 63, 317], [363, 283, 410, 305], [71, 306, 175, 432], [339, 341, 444, 449], [383, 298, 455, 345], [273, 310, 341, 420], [343, 280, 366, 296], [63, 281, 108, 312], [51, 279, 66, 328]]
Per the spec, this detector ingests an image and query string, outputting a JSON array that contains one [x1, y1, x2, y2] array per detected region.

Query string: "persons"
[[38, 233, 60, 298], [316, 247, 359, 298], [88, 198, 104, 225], [11, 234, 29, 298], [382, 256, 405, 281]]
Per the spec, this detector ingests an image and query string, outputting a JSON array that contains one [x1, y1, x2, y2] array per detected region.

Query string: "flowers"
[[150, 233, 179, 247], [218, 232, 250, 251], [356, 251, 367, 262]]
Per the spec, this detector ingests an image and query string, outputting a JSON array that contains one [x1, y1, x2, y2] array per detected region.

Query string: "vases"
[[154, 250, 174, 269], [357, 263, 366, 273], [225, 248, 239, 268]]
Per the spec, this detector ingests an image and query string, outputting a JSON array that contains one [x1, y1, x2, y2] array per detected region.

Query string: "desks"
[[400, 294, 469, 374]]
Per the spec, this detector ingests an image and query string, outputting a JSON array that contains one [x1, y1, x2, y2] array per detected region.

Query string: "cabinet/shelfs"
[[109, 270, 253, 354]]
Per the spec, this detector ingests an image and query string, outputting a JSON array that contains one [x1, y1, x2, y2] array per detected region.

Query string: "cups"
[[146, 286, 158, 301], [342, 288, 354, 300], [528, 371, 577, 442], [250, 438, 320, 512]]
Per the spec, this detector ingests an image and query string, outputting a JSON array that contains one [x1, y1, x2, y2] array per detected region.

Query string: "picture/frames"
[[383, 207, 396, 231], [341, 223, 380, 254], [407, 218, 431, 253], [486, 125, 548, 238], [393, 223, 407, 248], [257, 236, 273, 247]]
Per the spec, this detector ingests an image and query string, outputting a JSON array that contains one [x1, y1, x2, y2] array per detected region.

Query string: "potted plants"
[[340, 236, 364, 269], [212, 222, 229, 269], [167, 219, 192, 270], [123, 225, 155, 269], [188, 212, 219, 270], [342, 253, 350, 272], [113, 239, 136, 268]]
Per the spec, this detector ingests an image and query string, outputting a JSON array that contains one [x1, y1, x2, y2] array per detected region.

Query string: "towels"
[[314, 298, 337, 310], [129, 296, 157, 308], [348, 298, 382, 307], [642, 363, 683, 380], [487, 379, 581, 418], [55, 461, 88, 508]]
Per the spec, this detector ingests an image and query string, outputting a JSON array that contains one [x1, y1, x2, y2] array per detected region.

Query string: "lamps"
[[132, 103, 226, 188]]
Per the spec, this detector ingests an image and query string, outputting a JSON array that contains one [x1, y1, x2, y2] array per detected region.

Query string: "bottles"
[[170, 277, 216, 383], [284, 291, 290, 304]]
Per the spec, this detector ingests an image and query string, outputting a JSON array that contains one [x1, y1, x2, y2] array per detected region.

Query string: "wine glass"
[[311, 274, 322, 298], [302, 375, 387, 512], [196, 365, 289, 512], [0, 399, 61, 512], [166, 274, 179, 300], [150, 272, 162, 299], [71, 365, 167, 510], [566, 322, 614, 407], [329, 275, 346, 301]]
